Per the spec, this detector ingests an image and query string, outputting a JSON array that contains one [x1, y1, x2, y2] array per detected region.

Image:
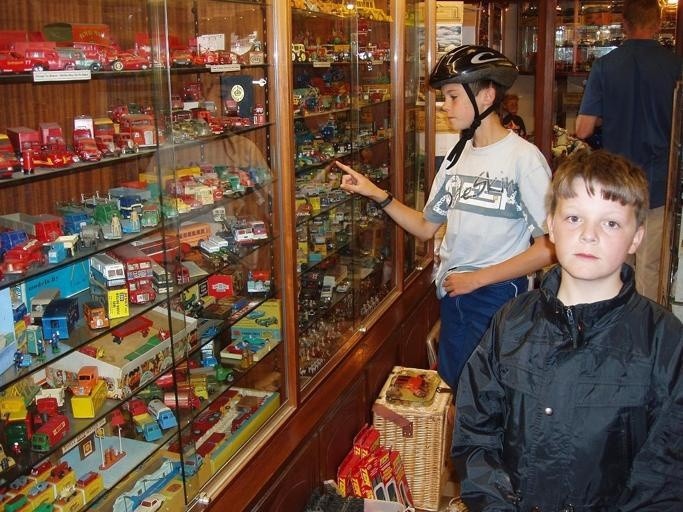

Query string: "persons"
[[334, 45, 555, 387], [452, 154, 682, 511], [575, 1, 681, 305]]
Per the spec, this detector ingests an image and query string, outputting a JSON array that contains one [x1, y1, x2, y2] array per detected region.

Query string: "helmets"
[[428, 45, 521, 92]]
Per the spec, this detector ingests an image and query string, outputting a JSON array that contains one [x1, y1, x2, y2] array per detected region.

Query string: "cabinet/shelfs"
[[0, 0, 438, 512], [517, 0, 678, 77]]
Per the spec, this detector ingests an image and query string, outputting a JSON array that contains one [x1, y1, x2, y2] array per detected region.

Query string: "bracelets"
[[381, 192, 393, 208]]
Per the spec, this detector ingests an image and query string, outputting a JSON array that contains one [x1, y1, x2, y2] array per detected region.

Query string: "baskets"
[[373, 365, 456, 512]]
